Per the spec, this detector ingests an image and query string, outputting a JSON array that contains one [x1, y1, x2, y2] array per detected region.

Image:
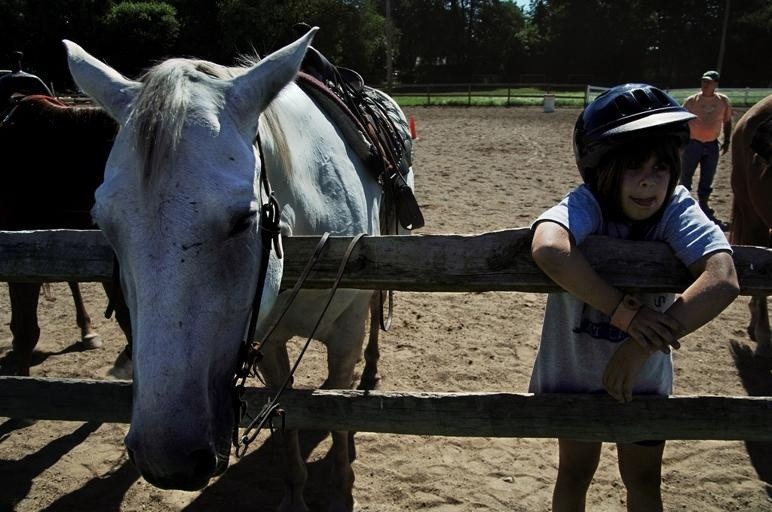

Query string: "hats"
[[702, 71, 720, 81]]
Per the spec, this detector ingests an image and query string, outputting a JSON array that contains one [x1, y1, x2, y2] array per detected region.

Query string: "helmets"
[[573, 83, 698, 190]]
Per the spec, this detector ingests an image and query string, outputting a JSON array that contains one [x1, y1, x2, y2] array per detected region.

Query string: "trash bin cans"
[[544, 94, 555, 113]]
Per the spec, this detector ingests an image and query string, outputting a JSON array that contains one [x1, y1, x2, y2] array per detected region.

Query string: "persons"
[[679, 69, 736, 232], [527, 83, 742, 512]]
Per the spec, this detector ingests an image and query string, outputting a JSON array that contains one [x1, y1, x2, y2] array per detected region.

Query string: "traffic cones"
[[409, 114, 419, 142]]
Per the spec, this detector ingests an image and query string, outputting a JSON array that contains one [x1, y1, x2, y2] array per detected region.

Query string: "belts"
[[695, 139, 717, 143]]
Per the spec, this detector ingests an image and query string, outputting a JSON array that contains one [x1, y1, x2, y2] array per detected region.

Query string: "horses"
[[3, 94, 132, 372], [728, 93, 772, 358], [62, 28, 415, 512]]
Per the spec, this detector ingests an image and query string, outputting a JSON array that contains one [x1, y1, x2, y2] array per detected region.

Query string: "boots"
[[699, 199, 715, 215]]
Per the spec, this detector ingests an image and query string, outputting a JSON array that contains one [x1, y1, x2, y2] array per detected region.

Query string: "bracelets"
[[610, 292, 644, 335]]
